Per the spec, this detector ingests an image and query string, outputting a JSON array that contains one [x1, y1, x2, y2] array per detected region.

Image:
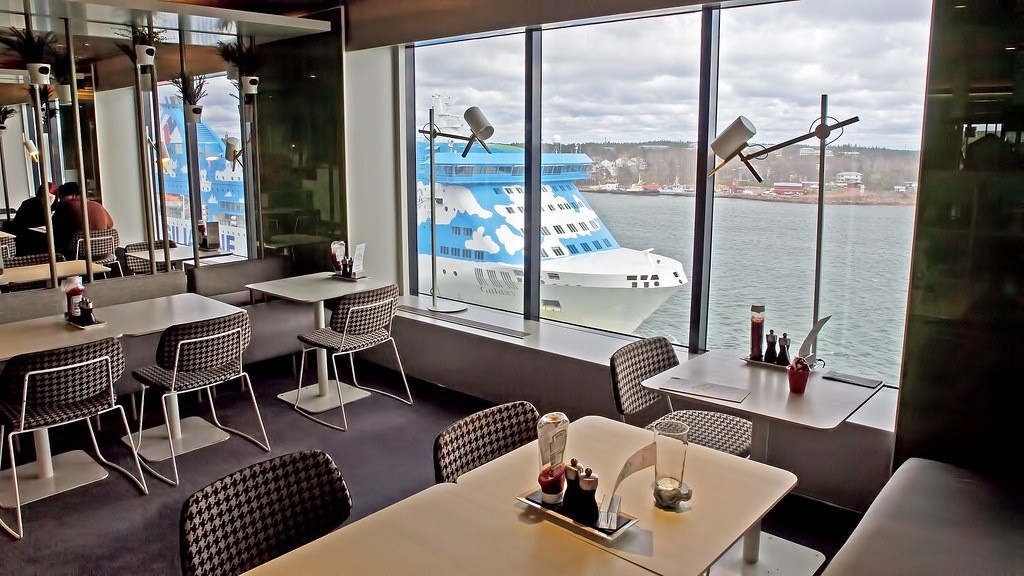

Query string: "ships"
[[141, 96, 689, 334]]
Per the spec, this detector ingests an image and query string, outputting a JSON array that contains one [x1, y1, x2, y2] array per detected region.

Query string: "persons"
[[11, 182, 59, 231], [58, 183, 113, 257]]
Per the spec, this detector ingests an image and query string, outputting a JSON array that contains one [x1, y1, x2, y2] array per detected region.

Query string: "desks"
[[27, 226, 57, 234], [0, 260, 112, 295], [259, 205, 304, 236], [0, 231, 16, 268], [249, 414, 798, 576], [0, 213, 16, 220], [258, 233, 332, 259], [246, 270, 395, 414], [0, 292, 248, 509], [125, 245, 234, 275], [640, 352, 886, 576]]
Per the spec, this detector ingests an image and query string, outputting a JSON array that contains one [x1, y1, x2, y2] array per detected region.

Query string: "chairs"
[[129, 311, 271, 486], [0, 237, 67, 276], [262, 208, 321, 261], [434, 400, 542, 487], [69, 228, 124, 280], [0, 337, 151, 540], [179, 448, 355, 576], [126, 239, 178, 276], [612, 336, 752, 464], [0, 209, 16, 231], [294, 283, 414, 431]]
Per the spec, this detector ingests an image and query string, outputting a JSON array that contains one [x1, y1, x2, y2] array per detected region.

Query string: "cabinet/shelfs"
[[314, 161, 342, 224]]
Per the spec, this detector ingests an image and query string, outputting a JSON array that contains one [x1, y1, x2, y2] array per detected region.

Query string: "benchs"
[[188, 253, 333, 398], [820, 314, 1024, 576], [3, 269, 186, 452]]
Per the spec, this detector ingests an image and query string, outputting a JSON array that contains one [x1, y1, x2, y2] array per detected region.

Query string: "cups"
[[786, 364, 810, 395], [749, 303, 766, 362], [653, 420, 690, 512]]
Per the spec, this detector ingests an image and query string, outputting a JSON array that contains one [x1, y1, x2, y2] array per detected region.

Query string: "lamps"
[[145, 126, 173, 240], [419, 105, 495, 314], [21, 132, 41, 199], [225, 135, 244, 172], [709, 93, 862, 384]]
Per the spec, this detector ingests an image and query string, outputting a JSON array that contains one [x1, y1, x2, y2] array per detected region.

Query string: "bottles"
[[341, 256, 353, 278], [78, 296, 96, 327], [578, 467, 600, 525], [776, 333, 790, 366], [331, 240, 346, 276], [536, 412, 570, 504], [197, 219, 206, 248], [66, 276, 86, 323], [764, 329, 777, 363], [561, 458, 583, 515]]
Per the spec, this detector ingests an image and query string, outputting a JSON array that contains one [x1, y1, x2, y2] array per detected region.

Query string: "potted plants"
[[112, 18, 167, 65], [216, 39, 268, 95], [237, 94, 254, 123], [171, 71, 208, 124], [0, 104, 19, 136], [1, 24, 58, 85], [54, 48, 82, 106]]
